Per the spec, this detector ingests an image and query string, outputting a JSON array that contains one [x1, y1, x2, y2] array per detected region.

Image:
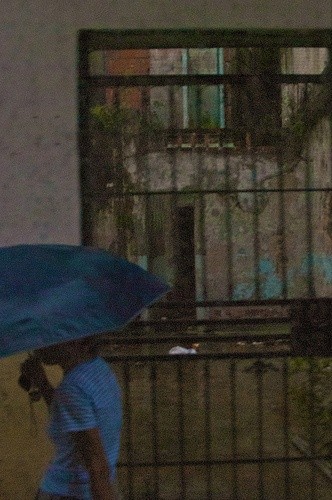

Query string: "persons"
[[19, 331, 126, 500]]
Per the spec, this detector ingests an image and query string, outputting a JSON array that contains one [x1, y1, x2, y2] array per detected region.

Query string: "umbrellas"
[[0, 244, 173, 402]]
